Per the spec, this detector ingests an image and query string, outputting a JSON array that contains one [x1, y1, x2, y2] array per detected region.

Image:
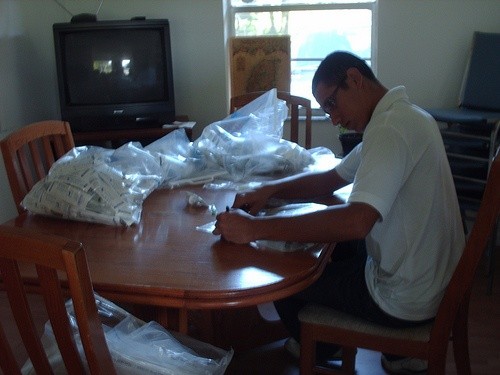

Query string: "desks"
[[0, 171, 338, 339]]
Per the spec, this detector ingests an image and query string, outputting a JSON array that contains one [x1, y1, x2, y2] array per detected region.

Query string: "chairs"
[[0, 91, 500, 375]]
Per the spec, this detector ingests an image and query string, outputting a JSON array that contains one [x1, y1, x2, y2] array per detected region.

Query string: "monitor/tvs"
[[52, 19, 178, 133]]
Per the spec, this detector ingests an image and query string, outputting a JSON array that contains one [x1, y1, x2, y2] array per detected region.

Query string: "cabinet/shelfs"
[[439, 119, 500, 203], [72, 115, 193, 147]]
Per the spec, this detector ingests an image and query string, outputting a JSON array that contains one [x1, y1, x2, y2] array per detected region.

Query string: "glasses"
[[323, 86, 340, 114]]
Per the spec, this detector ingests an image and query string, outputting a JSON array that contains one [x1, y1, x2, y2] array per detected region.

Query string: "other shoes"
[[380, 354, 429, 373], [285, 338, 358, 362]]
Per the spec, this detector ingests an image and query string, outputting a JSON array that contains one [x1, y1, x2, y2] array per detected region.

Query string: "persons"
[[211, 50, 466, 369]]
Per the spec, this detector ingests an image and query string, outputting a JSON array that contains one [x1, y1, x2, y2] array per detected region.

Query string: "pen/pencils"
[[226, 205, 229, 212]]
